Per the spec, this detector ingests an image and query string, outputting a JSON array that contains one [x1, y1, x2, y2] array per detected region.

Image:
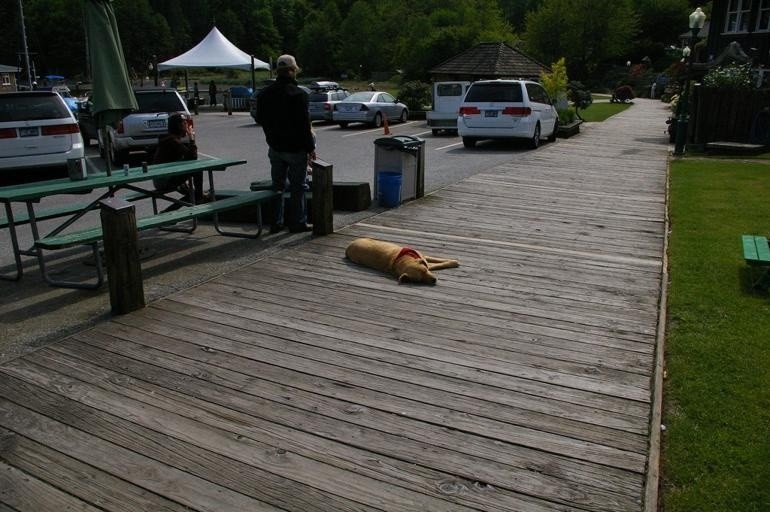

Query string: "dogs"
[[344, 237, 459, 287]]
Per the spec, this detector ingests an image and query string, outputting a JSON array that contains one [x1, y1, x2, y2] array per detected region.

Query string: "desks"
[[0, 157, 248, 283]]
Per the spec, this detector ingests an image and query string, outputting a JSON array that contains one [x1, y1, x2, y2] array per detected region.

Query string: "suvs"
[[74, 93, 99, 146], [96, 89, 198, 162], [307, 86, 352, 125], [0, 91, 87, 183]]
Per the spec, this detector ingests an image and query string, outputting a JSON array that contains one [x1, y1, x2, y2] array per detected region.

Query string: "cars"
[[332, 91, 410, 128], [223, 87, 253, 111]]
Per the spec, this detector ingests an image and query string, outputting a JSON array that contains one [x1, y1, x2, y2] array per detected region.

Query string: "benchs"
[[741, 231, 770, 293], [35, 190, 286, 289], [0, 189, 160, 280]]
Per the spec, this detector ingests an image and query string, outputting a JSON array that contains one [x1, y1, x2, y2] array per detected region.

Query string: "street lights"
[[626, 61, 631, 74], [672, 7, 706, 155], [674, 45, 691, 120], [148, 55, 158, 87]]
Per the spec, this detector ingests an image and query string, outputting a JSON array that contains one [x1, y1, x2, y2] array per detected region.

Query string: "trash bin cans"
[[373, 135, 426, 207]]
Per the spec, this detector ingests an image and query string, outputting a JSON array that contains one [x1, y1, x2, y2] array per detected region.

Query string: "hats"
[[277, 54, 300, 71]]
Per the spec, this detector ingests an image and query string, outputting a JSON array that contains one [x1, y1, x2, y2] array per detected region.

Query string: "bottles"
[[142, 161, 149, 173], [124, 164, 130, 176]]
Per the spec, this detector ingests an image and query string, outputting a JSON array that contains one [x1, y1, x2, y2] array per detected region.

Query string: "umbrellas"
[[81, 1, 139, 198]]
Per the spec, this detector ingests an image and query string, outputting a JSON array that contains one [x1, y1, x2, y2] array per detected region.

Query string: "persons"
[[209, 79, 218, 109], [248, 54, 316, 233], [63, 91, 79, 110], [152, 113, 204, 205], [193, 81, 199, 97]]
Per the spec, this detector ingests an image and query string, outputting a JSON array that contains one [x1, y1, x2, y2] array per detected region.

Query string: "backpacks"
[[145, 136, 176, 165]]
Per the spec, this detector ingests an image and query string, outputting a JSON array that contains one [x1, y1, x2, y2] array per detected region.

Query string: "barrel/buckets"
[[376, 170, 402, 206]]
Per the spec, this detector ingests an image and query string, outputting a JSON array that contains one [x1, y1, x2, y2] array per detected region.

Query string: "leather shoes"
[[195, 189, 213, 204], [293, 223, 313, 234]]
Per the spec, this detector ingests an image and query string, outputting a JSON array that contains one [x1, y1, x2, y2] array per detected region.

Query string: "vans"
[[456, 78, 559, 150]]
